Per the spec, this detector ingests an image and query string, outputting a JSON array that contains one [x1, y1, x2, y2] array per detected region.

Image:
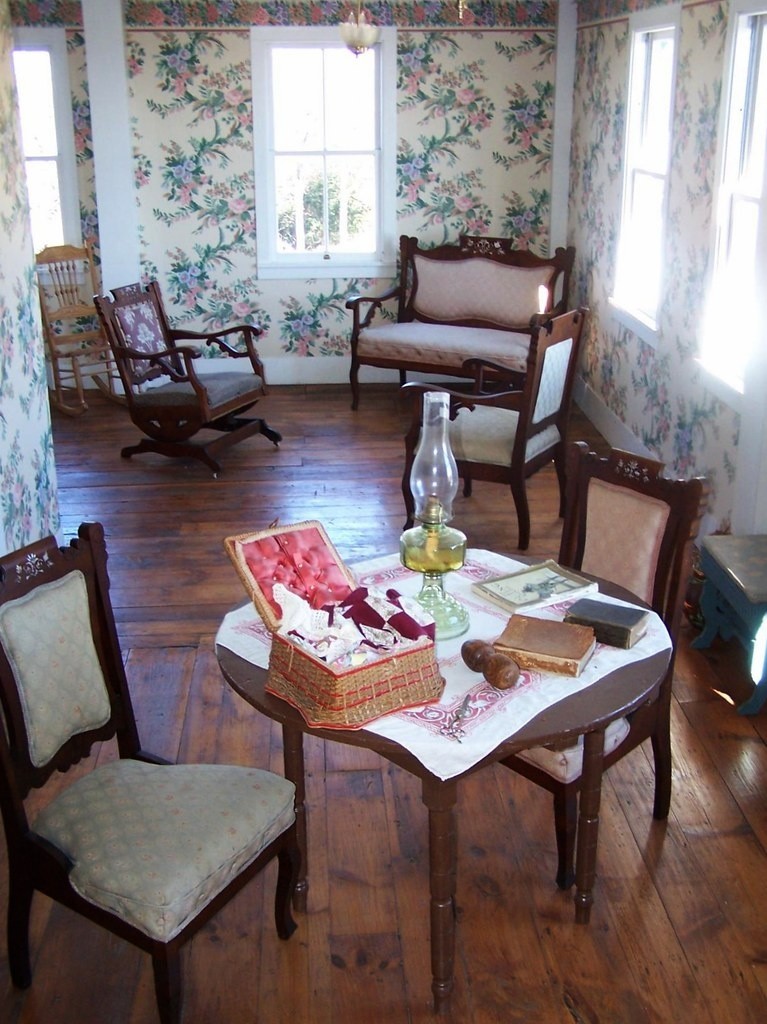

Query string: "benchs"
[[344, 233, 577, 412]]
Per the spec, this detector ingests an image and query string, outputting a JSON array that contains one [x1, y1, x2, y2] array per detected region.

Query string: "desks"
[[214, 556, 673, 1018]]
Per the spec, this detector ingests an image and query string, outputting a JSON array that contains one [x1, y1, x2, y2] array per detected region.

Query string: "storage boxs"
[[222, 517, 446, 732]]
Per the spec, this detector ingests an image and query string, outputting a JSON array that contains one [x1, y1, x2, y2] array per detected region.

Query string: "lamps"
[[338, 0, 382, 57], [399, 389, 470, 640]]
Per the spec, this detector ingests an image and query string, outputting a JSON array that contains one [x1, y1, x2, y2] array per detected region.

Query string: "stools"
[[691, 534, 767, 716]]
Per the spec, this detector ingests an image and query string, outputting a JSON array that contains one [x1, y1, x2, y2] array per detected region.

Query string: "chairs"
[[92, 281, 283, 479], [400, 303, 591, 551], [0, 520, 304, 1024], [495, 437, 713, 888], [35, 239, 130, 417]]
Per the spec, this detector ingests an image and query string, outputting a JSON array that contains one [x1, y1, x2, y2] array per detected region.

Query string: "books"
[[472, 558, 599, 614], [563, 598, 649, 651], [493, 613, 597, 678]]
